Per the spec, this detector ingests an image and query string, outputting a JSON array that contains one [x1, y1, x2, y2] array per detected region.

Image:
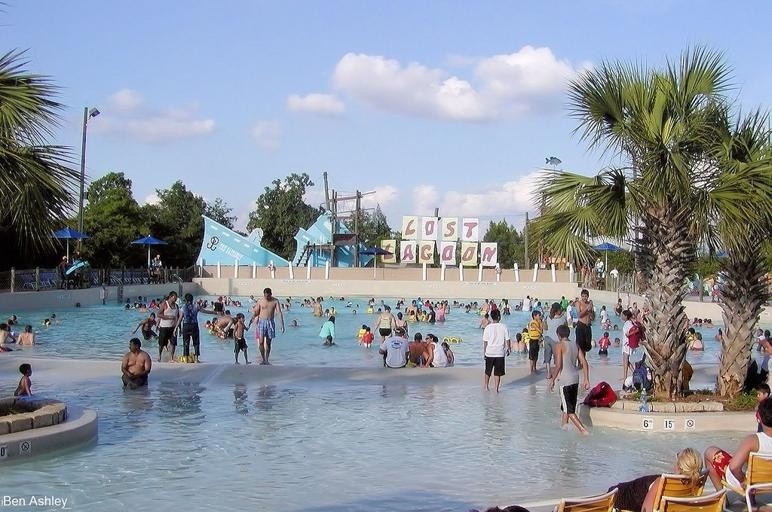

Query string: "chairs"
[[20, 270, 148, 290], [553, 487, 619, 512], [661, 488, 727, 512], [721, 451, 772, 512], [652, 468, 711, 512]]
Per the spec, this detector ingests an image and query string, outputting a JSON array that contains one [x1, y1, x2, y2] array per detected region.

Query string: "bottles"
[[639, 388, 649, 412]]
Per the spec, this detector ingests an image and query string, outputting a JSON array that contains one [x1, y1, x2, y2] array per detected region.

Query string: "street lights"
[[77, 107, 102, 270]]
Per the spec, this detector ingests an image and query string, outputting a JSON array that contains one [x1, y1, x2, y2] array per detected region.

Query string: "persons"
[[1, 314, 56, 351], [149, 255, 166, 285], [747, 324, 771, 427], [56, 253, 93, 283], [356, 298, 515, 369], [15, 364, 32, 398], [549, 325, 590, 434], [704, 398, 772, 508], [577, 290, 594, 365], [583, 257, 618, 292], [685, 318, 724, 353], [483, 310, 511, 393], [516, 296, 578, 374], [641, 449, 703, 511], [599, 298, 647, 387], [122, 287, 356, 386]]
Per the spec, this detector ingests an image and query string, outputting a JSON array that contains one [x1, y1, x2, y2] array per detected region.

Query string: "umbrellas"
[[360, 245, 393, 276], [590, 243, 623, 272], [711, 252, 729, 258], [48, 227, 92, 276], [131, 234, 168, 269]]
